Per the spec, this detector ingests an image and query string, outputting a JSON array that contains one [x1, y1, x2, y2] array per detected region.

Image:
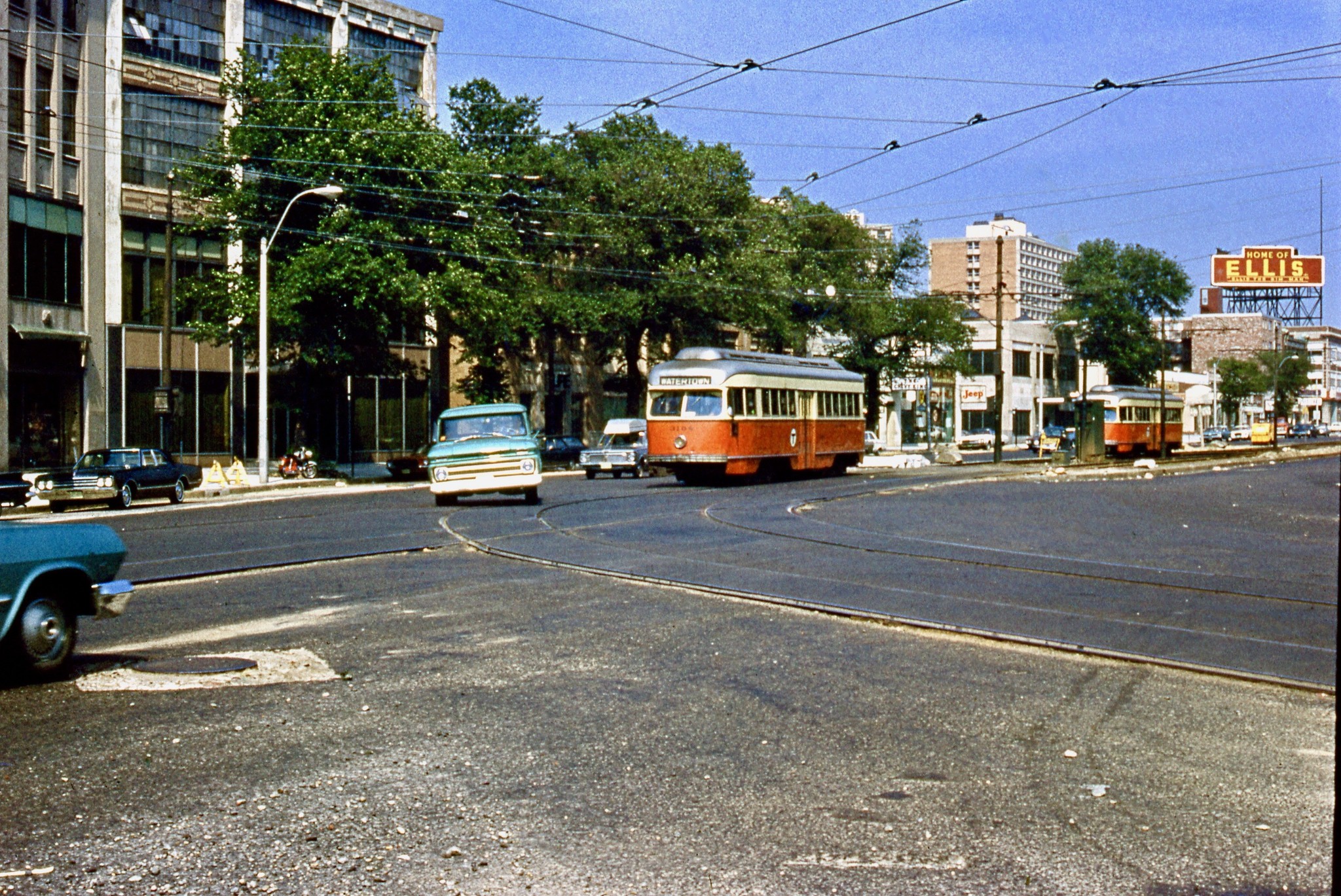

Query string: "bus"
[[644, 347, 866, 482], [1076, 384, 1184, 460]]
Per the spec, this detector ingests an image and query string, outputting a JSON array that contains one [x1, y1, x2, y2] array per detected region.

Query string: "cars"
[[0, 517, 135, 680], [0, 470, 36, 517], [537, 435, 590, 472], [864, 431, 886, 457], [1025, 426, 1077, 454], [1203, 417, 1341, 444], [916, 426, 1008, 451], [33, 447, 204, 512], [386, 441, 435, 482]]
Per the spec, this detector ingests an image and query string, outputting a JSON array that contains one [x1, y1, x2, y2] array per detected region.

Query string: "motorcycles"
[[278, 444, 319, 479]]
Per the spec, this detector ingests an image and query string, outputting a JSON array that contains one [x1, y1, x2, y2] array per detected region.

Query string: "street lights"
[[1274, 355, 1300, 450], [257, 185, 344, 483], [1038, 319, 1079, 432], [1213, 346, 1241, 427]]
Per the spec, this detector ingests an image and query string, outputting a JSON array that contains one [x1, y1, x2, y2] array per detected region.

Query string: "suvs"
[[424, 403, 546, 506], [579, 418, 674, 479]]
